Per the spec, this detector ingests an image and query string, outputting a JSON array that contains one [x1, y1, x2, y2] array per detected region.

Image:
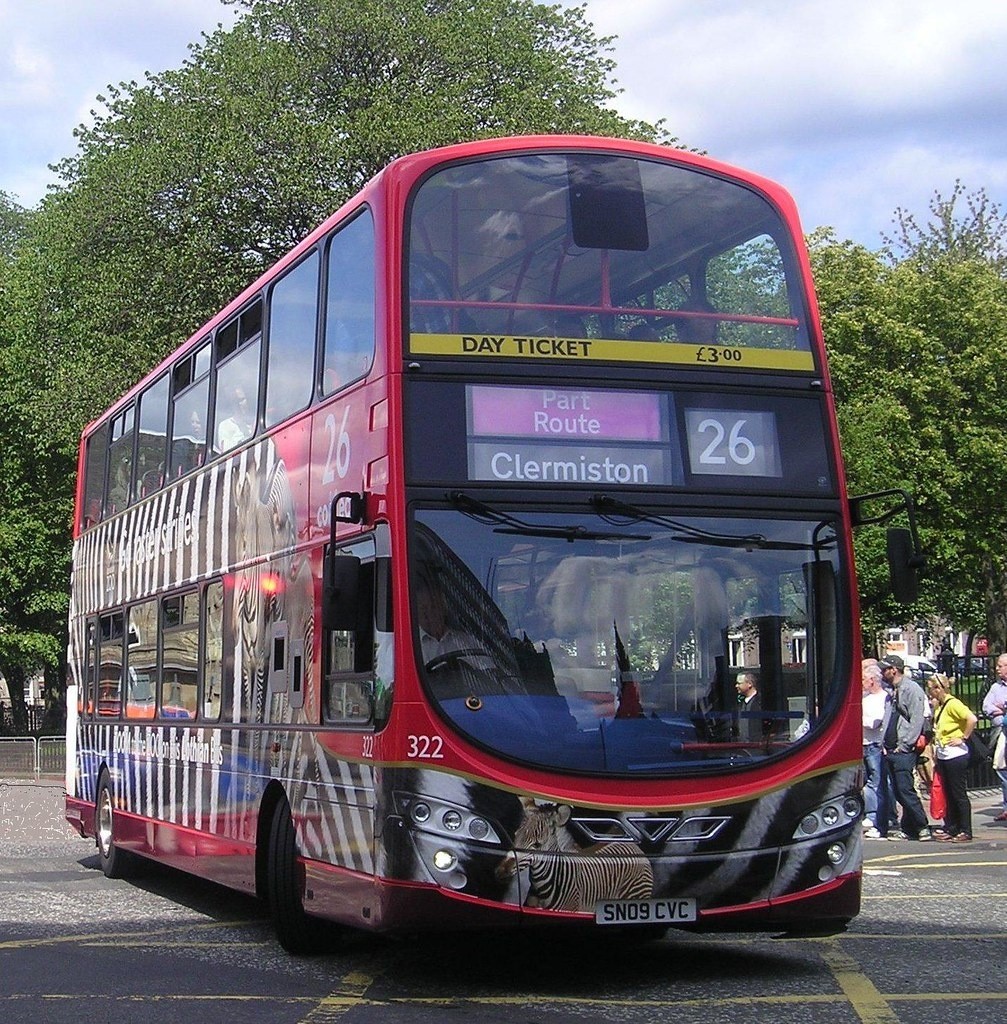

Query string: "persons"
[[735, 670, 762, 741], [675, 298, 718, 344], [983, 653, 1007, 821], [861, 654, 978, 842]]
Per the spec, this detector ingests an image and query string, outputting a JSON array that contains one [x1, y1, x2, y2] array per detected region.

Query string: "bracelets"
[[963, 735, 968, 740]]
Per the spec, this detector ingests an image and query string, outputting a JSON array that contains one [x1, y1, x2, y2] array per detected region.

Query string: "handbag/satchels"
[[965, 731, 989, 771], [929, 769, 948, 820], [921, 719, 932, 743]]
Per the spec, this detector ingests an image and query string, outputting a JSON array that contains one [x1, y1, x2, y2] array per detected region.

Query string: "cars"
[[905, 655, 989, 677]]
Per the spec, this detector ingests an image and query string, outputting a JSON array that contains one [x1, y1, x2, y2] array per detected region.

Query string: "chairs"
[[195, 441, 204, 466], [157, 462, 166, 486], [87, 498, 101, 528], [142, 469, 159, 497]]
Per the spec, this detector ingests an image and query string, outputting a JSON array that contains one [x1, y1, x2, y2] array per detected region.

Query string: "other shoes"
[[918, 828, 932, 841], [864, 827, 880, 837], [994, 811, 1007, 821], [932, 829, 951, 838], [887, 831, 909, 841], [862, 818, 874, 827], [946, 831, 972, 843]]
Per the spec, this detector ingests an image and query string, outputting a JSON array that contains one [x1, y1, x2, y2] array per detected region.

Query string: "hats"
[[877, 655, 905, 670]]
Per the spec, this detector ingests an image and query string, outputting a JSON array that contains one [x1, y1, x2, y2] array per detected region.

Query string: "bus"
[[65, 135, 926, 957]]
